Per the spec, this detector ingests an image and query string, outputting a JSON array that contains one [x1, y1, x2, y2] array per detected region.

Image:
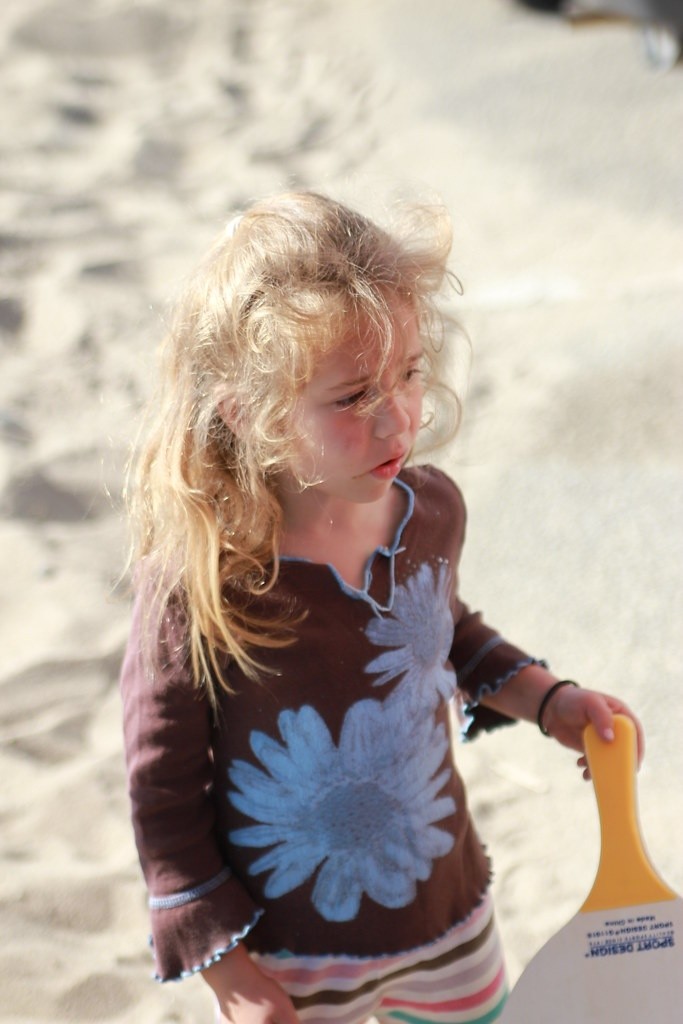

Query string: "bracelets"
[[538, 680, 580, 737]]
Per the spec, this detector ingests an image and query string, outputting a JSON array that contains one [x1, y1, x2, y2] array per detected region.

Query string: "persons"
[[120, 188, 646, 1023]]
